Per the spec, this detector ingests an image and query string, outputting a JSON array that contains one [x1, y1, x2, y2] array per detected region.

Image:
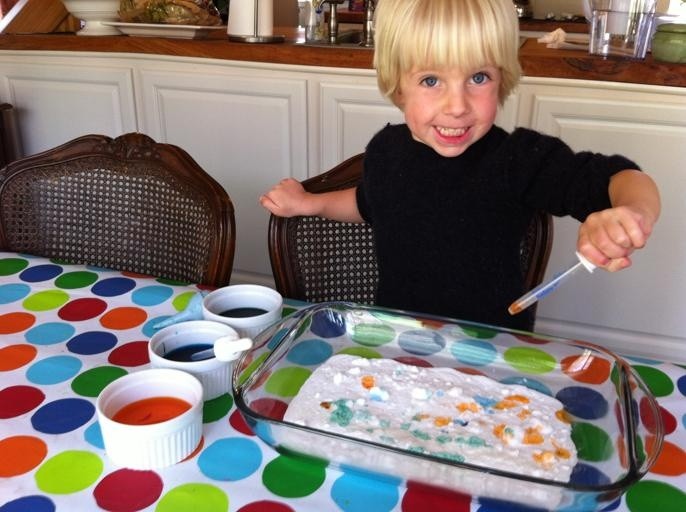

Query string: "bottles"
[[304, 0, 325, 46]]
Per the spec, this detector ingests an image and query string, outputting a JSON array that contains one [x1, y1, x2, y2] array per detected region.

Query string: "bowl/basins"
[[95, 283, 284, 470]]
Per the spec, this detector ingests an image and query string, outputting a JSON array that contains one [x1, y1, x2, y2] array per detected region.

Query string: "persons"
[[259, 1, 661, 332]]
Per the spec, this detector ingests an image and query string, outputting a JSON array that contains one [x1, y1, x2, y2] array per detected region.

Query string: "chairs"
[[5, 132, 237, 291], [2, 102, 24, 162], [268, 149, 551, 336]]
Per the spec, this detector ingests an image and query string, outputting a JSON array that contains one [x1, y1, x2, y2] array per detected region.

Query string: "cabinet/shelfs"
[[1, 51, 684, 367]]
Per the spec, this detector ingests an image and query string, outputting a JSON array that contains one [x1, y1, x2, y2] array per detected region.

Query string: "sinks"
[[311, 29, 529, 49]]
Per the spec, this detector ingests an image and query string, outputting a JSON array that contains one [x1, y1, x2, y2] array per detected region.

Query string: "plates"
[[101, 21, 227, 39]]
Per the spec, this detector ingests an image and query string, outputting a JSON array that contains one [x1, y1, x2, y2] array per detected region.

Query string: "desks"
[[1, 248, 685, 512]]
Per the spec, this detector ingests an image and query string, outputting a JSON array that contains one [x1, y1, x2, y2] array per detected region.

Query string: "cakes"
[[283, 352, 577, 509]]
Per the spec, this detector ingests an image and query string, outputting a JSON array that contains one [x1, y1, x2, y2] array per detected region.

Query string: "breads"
[[122, 0, 222, 26]]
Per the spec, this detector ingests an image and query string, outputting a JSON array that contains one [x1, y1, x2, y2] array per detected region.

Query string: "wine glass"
[[60, 1, 123, 35]]
